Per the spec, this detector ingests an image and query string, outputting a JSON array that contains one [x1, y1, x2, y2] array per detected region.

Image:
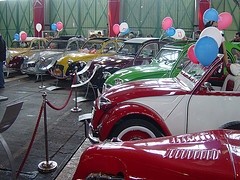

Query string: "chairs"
[[221, 63, 240, 91], [0, 101, 24, 180]]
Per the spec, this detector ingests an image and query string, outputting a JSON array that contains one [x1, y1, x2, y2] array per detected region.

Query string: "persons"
[[231, 32, 240, 41], [0, 34, 6, 89]]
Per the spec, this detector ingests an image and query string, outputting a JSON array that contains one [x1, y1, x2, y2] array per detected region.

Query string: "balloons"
[[218, 12, 232, 30], [113, 24, 120, 35], [198, 27, 221, 48], [19, 31, 27, 40], [171, 29, 185, 39], [120, 23, 128, 32], [35, 23, 42, 32], [121, 29, 130, 35], [162, 18, 172, 30], [14, 34, 20, 40], [57, 22, 63, 31], [166, 27, 175, 36], [51, 23, 57, 31], [188, 45, 199, 64], [203, 8, 219, 26], [194, 36, 218, 66]]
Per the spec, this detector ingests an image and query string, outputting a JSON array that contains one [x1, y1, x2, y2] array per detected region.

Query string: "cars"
[[3, 32, 240, 97], [71, 129, 240, 180], [86, 49, 240, 144]]
